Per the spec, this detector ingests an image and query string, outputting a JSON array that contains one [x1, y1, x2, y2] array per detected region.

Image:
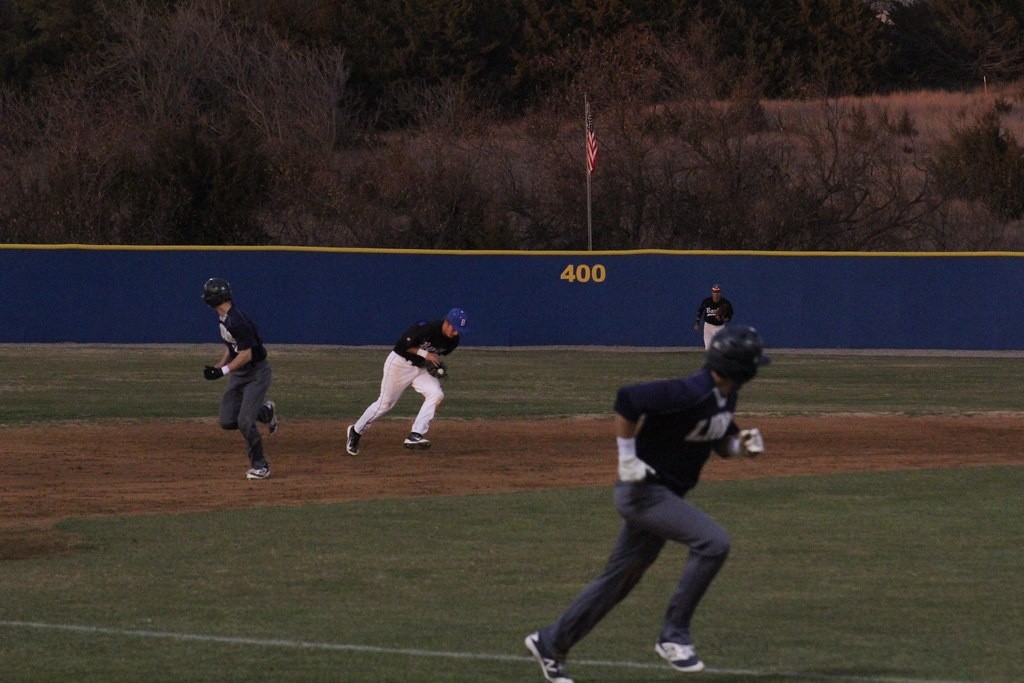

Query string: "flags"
[[587, 108, 599, 174]]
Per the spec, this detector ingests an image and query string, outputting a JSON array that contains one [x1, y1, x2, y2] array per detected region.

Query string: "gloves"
[[740, 428, 764, 458], [617, 456, 656, 483], [203, 365, 224, 381]]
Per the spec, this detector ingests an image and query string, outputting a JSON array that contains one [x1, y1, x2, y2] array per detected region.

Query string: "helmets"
[[201, 278, 231, 302], [709, 325, 771, 382], [447, 307, 470, 334]]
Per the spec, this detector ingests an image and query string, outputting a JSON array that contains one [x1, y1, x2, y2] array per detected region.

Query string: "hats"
[[712, 285, 721, 293]]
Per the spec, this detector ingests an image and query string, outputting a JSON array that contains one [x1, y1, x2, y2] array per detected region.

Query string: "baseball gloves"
[[424, 361, 450, 380]]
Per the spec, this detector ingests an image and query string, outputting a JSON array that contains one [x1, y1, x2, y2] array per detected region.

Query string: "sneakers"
[[404, 434, 431, 449], [347, 425, 362, 455], [246, 463, 270, 479], [525, 632, 574, 683], [265, 401, 279, 434], [654, 637, 704, 673]]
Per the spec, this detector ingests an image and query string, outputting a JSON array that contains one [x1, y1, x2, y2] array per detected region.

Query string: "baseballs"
[[437, 368, 444, 375]]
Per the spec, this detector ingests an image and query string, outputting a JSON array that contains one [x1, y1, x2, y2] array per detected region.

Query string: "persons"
[[525, 327, 772, 683], [201, 278, 278, 480], [694, 283, 733, 350], [346, 308, 469, 457]]
